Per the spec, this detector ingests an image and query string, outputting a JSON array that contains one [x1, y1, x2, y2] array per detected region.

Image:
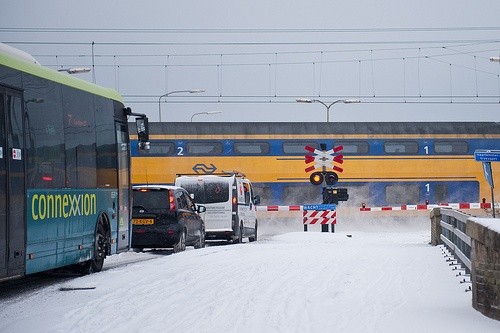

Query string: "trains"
[[126, 122, 500, 234]]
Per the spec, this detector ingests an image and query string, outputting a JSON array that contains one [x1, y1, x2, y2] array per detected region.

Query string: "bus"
[[0, 43, 150, 281]]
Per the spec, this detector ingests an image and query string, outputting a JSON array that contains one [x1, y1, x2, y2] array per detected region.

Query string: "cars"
[[131, 185, 206, 252]]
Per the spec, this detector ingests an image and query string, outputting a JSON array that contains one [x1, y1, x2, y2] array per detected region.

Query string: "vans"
[[175, 171, 258, 242]]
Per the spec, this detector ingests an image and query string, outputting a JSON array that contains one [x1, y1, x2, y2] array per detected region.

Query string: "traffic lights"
[[310, 171, 339, 186]]
